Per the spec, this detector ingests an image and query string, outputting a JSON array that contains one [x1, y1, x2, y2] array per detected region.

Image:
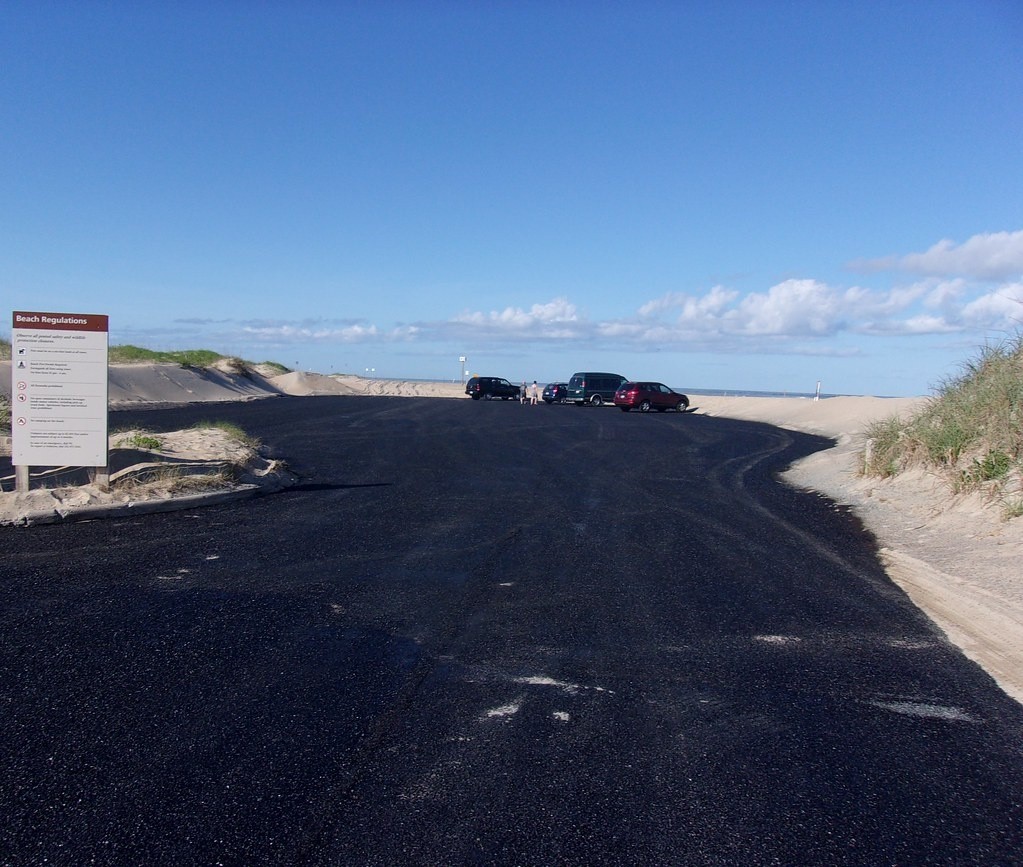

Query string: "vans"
[[566, 372, 629, 407]]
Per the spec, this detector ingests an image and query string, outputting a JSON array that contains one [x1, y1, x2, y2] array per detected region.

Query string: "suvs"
[[542, 382, 569, 405], [615, 382, 689, 413], [465, 377, 520, 401]]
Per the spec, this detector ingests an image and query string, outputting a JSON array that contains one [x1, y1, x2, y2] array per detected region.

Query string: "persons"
[[520, 381, 528, 405], [529, 380, 539, 405]]
